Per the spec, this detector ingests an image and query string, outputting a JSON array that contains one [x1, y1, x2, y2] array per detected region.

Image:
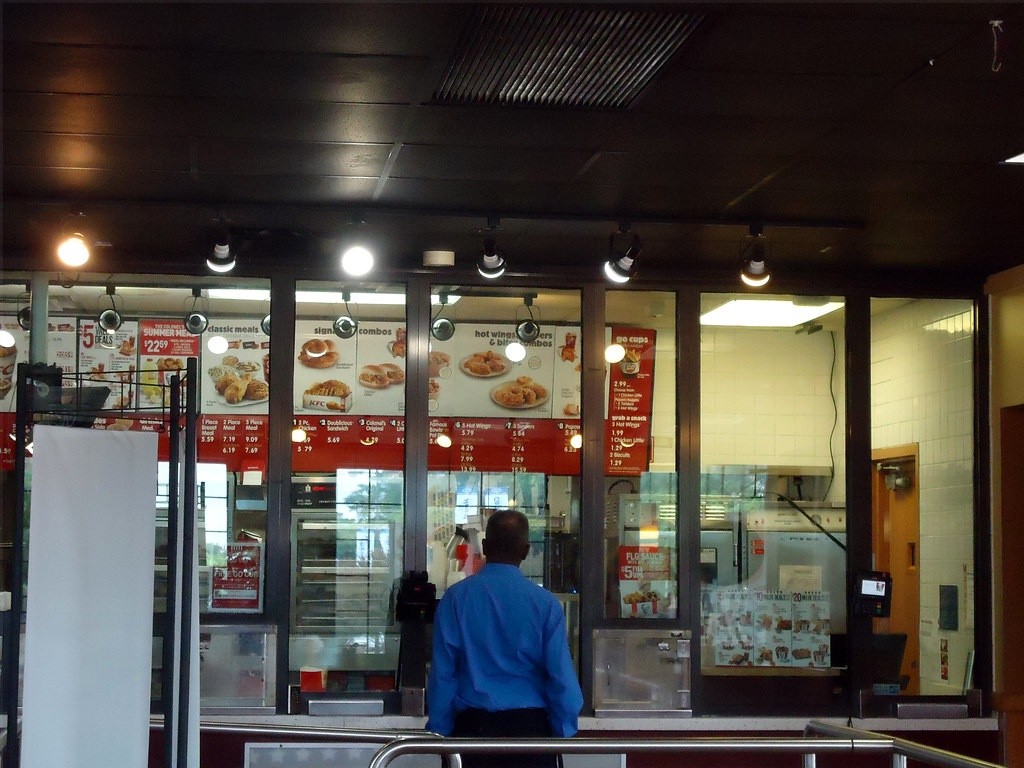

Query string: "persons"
[[426, 510, 584, 768]]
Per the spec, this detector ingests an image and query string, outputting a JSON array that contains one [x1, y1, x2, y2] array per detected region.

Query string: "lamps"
[[98, 286, 124, 332], [603, 220, 644, 283], [261, 314, 270, 336], [739, 221, 774, 288], [16, 292, 32, 330], [57, 210, 89, 266], [182, 287, 211, 334], [478, 222, 507, 279], [431, 292, 457, 342], [515, 294, 541, 343], [334, 288, 359, 338], [207, 225, 236, 272]]
